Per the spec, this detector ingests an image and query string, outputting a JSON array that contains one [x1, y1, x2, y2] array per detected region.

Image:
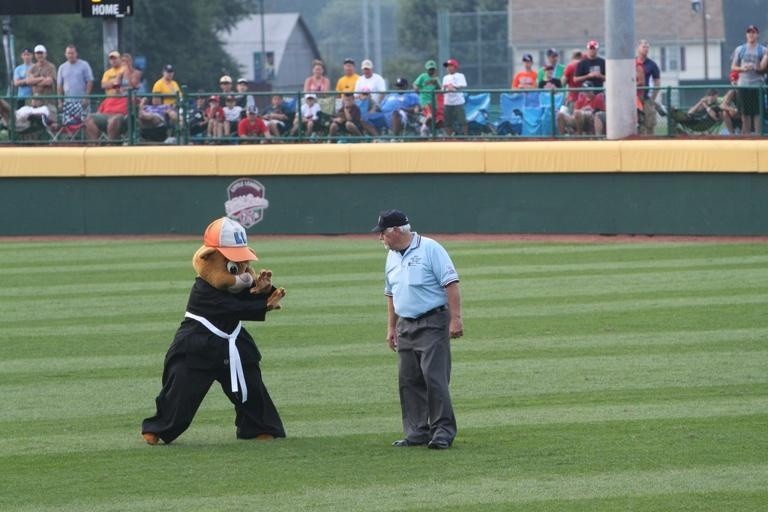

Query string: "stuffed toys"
[[138, 215, 288, 446]]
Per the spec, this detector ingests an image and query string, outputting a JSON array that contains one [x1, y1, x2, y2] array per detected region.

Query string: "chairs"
[[464, 91, 566, 137], [673, 95, 728, 135], [0, 112, 47, 141], [41, 101, 87, 145]]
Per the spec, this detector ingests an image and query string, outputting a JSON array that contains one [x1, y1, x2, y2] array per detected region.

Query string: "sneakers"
[[393, 439, 422, 446], [428, 440, 450, 448]]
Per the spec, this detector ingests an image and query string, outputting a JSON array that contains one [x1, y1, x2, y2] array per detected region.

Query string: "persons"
[[512, 41, 606, 137], [671, 25, 768, 135], [635, 40, 661, 136], [368, 207, 464, 450], [2, 44, 468, 143]]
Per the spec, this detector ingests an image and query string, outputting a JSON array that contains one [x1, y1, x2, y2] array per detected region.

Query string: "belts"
[[418, 305, 448, 319]]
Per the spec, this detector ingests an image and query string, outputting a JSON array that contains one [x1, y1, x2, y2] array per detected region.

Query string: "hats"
[[362, 58, 374, 70], [523, 48, 561, 74], [745, 25, 760, 34], [107, 50, 121, 59], [425, 60, 437, 70], [219, 75, 233, 84], [162, 63, 176, 74], [236, 78, 249, 84], [394, 78, 409, 87], [370, 209, 410, 235], [203, 216, 259, 264], [443, 59, 459, 67], [33, 43, 47, 53], [586, 40, 600, 50]]
